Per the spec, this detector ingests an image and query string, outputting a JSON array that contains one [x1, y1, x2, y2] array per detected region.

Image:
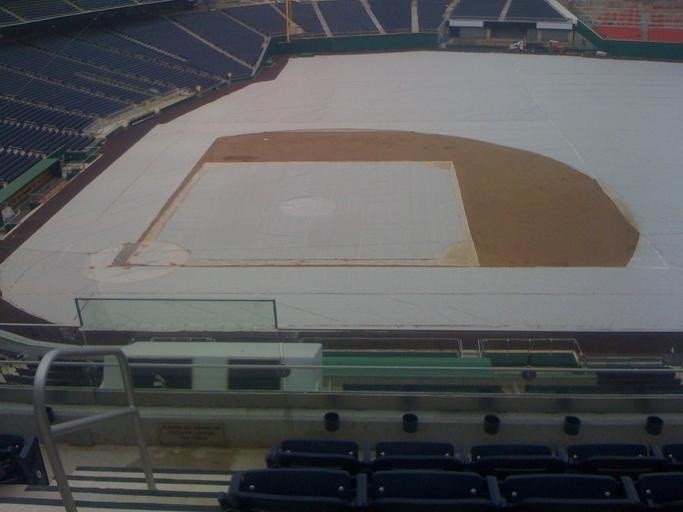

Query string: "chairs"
[[0, 50, 72, 242], [220, 344, 682, 512], [2, 354, 104, 488], [73, 50, 263, 151]]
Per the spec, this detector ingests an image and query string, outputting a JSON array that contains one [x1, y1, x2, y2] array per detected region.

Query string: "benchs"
[[1, 0, 682, 50]]
